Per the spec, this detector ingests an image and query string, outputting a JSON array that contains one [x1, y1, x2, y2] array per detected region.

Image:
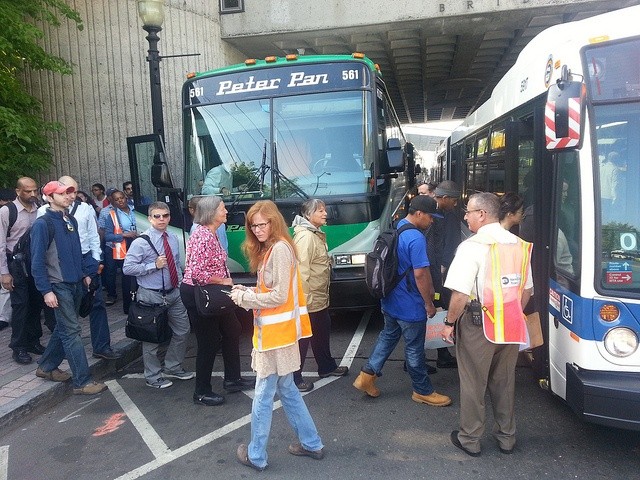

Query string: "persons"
[[27, 180, 110, 396], [289, 197, 349, 391], [187, 195, 230, 260], [90, 183, 111, 219], [230, 198, 326, 472], [0, 176, 46, 364], [103, 189, 139, 315], [122, 181, 150, 212], [0, 188, 17, 330], [416, 182, 438, 202], [497, 191, 527, 230], [199, 144, 251, 201], [560, 178, 570, 206], [439, 191, 535, 457], [598, 151, 627, 223], [34, 174, 123, 361], [76, 189, 104, 219], [179, 193, 255, 407], [351, 194, 454, 407], [96, 186, 121, 307], [121, 199, 195, 391], [403, 179, 463, 374]]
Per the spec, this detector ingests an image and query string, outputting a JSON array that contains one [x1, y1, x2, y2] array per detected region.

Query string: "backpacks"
[[364, 217, 422, 301], [7, 214, 77, 311]]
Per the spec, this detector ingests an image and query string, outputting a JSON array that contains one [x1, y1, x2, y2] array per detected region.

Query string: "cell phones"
[[220, 289, 231, 296], [470, 298, 482, 325]]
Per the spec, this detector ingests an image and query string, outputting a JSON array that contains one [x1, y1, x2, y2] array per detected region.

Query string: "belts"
[[149, 288, 178, 295]]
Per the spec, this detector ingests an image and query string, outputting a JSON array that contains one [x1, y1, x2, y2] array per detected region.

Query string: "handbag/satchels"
[[195, 284, 238, 318], [126, 302, 168, 344]]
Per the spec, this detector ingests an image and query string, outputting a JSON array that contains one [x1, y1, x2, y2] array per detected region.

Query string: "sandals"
[[237, 444, 268, 472], [288, 443, 323, 459]]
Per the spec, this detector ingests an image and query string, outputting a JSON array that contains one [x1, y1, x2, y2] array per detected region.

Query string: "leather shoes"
[[193, 390, 224, 405], [29, 344, 45, 354], [12, 349, 32, 364], [223, 377, 256, 392]]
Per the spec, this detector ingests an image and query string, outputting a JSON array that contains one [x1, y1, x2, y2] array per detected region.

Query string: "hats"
[[409, 194, 444, 219], [43, 181, 75, 196], [106, 188, 118, 196]]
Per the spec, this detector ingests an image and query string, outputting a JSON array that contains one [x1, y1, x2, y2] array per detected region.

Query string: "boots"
[[412, 392, 451, 406], [353, 372, 380, 397]]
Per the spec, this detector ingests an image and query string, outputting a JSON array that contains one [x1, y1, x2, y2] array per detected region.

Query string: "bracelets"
[[443, 317, 459, 327]]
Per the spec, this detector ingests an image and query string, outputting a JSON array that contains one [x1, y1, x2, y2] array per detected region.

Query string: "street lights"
[[139, 3, 167, 168]]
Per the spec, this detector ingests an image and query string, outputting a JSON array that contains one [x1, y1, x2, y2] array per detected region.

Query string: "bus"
[[127, 54, 421, 314], [436, 6, 640, 428]]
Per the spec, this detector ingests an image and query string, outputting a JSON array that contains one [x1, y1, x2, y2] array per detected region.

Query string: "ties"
[[162, 231, 178, 290]]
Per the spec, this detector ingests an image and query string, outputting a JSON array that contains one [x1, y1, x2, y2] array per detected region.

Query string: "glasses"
[[149, 213, 171, 219], [91, 188, 103, 191], [516, 210, 526, 219]]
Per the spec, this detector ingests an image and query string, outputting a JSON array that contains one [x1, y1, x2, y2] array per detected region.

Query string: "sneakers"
[[450, 430, 481, 458], [92, 349, 122, 360], [36, 365, 73, 382], [73, 382, 108, 397], [436, 357, 458, 369], [162, 367, 193, 380], [404, 362, 437, 374], [105, 300, 118, 305], [499, 444, 512, 454], [145, 377, 172, 388], [295, 380, 314, 392], [319, 367, 349, 378]]
